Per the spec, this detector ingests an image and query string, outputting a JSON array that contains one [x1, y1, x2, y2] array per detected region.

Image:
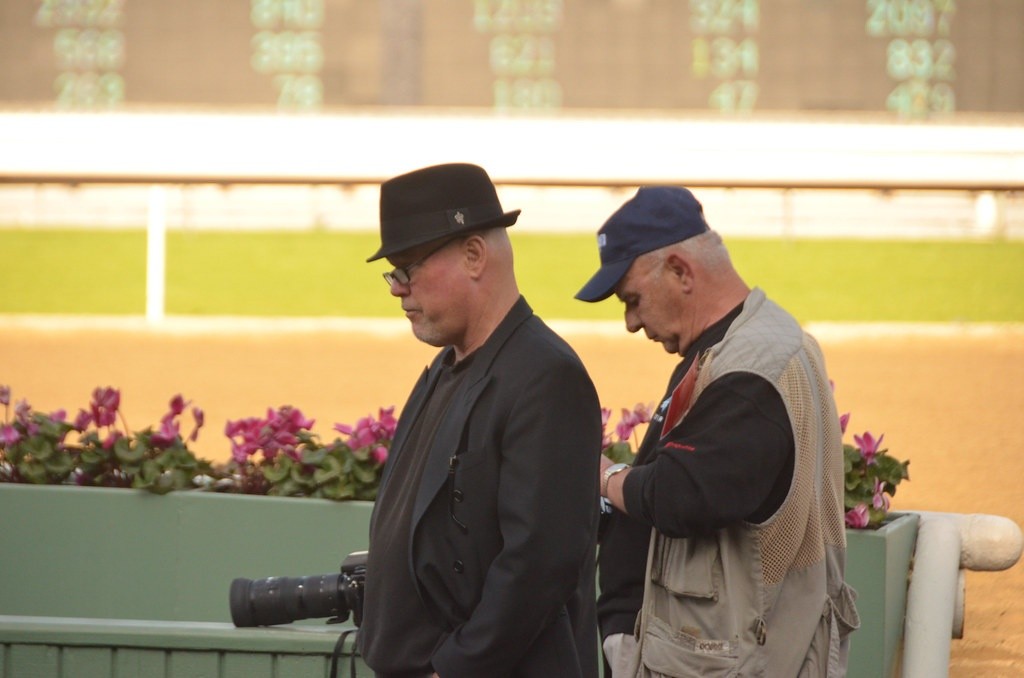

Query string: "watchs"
[[602, 463, 630, 506]]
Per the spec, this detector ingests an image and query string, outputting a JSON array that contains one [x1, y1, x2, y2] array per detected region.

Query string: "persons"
[[361, 163, 603, 678], [575, 186, 859, 677]]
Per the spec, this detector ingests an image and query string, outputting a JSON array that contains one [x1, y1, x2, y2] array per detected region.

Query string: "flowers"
[[0, 381, 910, 530]]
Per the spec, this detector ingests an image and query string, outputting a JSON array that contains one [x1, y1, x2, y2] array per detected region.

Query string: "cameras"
[[229, 550, 369, 628]]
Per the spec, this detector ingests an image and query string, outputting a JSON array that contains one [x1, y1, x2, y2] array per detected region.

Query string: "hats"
[[574, 186, 711, 302], [366, 162, 521, 263]]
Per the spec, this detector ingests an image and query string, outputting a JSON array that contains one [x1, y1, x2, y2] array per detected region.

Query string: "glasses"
[[383, 234, 485, 286]]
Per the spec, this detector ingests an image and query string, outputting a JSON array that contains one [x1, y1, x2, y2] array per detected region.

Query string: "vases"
[[0, 483, 919, 678]]
